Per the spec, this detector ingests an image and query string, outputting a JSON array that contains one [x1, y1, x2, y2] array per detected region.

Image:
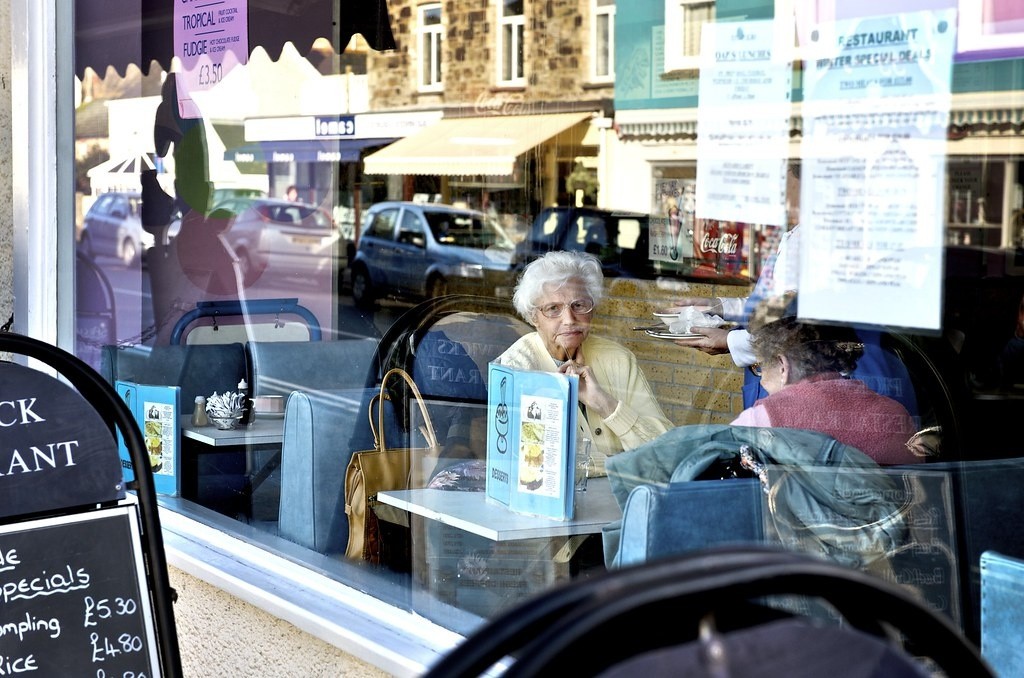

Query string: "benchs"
[[246, 388, 411, 591], [609, 459, 1024, 659]]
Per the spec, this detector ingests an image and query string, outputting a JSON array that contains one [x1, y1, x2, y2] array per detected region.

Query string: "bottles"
[[237, 379, 255, 425], [191, 396, 208, 427]]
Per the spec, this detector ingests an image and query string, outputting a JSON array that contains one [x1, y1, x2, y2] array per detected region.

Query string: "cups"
[[668, 207, 683, 260], [253, 395, 284, 413]]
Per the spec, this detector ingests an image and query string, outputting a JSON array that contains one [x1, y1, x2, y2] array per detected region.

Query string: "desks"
[[376, 476, 626, 582], [183, 406, 286, 514]]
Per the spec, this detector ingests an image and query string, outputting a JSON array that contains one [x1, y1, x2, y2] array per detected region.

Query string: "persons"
[[287, 186, 299, 201], [662, 157, 920, 429], [729, 289, 924, 465], [494, 250, 675, 580]]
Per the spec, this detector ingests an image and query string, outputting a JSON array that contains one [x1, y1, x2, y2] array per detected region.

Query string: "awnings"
[[363, 112, 592, 176], [74, 0, 396, 81], [223, 138, 399, 162]]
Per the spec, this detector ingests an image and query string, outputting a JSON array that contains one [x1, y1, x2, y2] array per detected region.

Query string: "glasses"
[[531, 298, 595, 318], [749, 361, 765, 376]]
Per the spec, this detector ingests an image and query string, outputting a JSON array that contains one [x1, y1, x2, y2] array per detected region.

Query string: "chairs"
[[100, 336, 252, 526], [277, 212, 294, 224], [244, 339, 383, 537]]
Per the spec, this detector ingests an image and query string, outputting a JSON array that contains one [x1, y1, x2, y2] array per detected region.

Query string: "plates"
[[255, 413, 284, 419], [645, 322, 739, 339]]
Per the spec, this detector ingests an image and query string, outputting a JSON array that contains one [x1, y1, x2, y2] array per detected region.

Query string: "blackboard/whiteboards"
[[327, 294, 538, 613], [0, 330, 185, 677], [73, 256, 119, 388], [417, 549, 1004, 678]]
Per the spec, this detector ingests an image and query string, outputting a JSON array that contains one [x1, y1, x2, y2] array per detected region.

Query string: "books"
[[486, 363, 579, 521]]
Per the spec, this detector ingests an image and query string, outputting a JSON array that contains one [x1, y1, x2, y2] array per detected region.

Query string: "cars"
[[509, 204, 659, 296], [210, 198, 349, 296], [352, 200, 518, 310], [78, 191, 183, 270]]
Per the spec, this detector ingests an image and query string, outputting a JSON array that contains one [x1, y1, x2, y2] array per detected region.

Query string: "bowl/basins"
[[652, 305, 714, 327]]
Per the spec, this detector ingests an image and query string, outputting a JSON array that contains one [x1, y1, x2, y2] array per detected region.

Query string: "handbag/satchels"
[[346, 368, 446, 569]]
